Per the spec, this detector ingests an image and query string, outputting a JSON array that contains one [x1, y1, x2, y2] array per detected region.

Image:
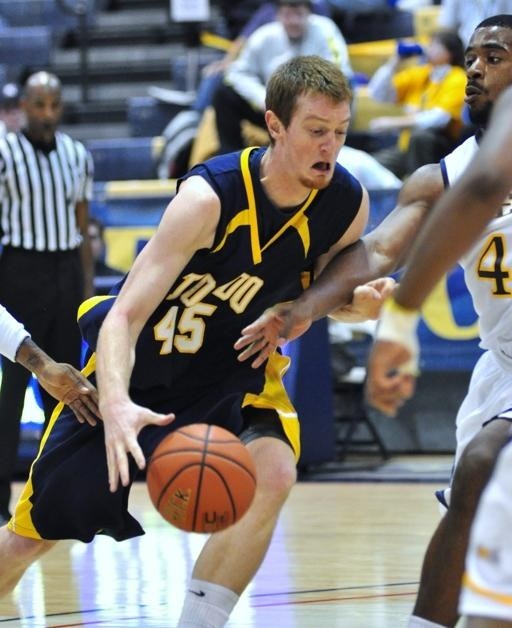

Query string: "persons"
[[1, 304, 103, 426], [366, 91, 511, 415], [0, 73, 95, 525], [213, 1, 512, 154]]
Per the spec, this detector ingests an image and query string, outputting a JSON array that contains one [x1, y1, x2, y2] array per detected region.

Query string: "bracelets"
[[375, 300, 420, 345]]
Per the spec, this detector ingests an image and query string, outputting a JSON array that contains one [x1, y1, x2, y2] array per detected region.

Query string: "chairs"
[[327, 320, 389, 463]]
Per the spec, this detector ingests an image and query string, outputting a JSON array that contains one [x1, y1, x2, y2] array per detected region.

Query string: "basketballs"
[[147, 423, 256, 533]]
